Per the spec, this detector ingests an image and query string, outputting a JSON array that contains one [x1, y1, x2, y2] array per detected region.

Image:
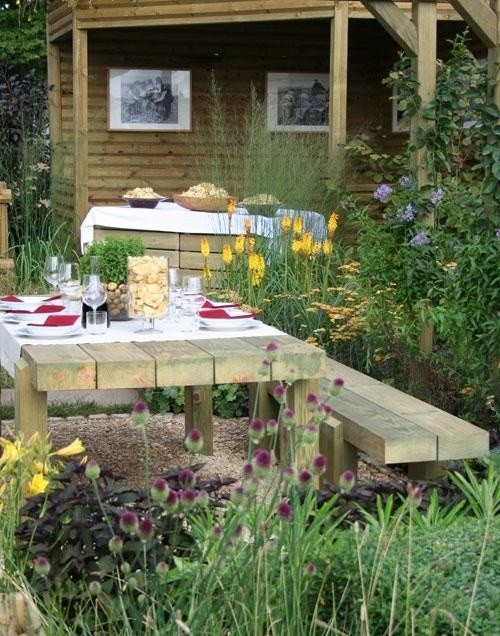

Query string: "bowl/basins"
[[119, 195, 168, 209], [238, 200, 282, 218]]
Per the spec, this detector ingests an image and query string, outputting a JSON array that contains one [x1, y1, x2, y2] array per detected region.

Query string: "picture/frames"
[[106, 64, 194, 134], [263, 69, 333, 132], [387, 69, 412, 134]]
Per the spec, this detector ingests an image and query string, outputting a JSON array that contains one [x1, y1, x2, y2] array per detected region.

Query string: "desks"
[[84, 201, 327, 309], [0, 292, 327, 503]]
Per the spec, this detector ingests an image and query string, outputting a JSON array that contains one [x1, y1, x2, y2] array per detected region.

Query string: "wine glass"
[[43, 254, 107, 332], [126, 255, 167, 336]]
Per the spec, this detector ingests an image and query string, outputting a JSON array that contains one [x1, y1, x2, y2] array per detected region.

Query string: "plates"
[[198, 298, 263, 331], [0, 296, 85, 338]]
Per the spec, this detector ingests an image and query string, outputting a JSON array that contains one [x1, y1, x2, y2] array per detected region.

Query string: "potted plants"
[[78, 234, 149, 321]]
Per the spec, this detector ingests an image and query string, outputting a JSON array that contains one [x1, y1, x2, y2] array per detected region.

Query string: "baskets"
[[173, 193, 234, 213]]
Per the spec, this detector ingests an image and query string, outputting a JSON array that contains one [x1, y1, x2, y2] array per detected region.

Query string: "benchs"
[[248, 354, 491, 521]]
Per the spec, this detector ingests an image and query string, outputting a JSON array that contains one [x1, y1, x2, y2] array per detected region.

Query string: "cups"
[[85, 310, 107, 339], [166, 266, 206, 334]]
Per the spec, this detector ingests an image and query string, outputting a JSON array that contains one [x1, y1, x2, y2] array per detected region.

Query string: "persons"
[[122, 74, 176, 122], [279, 76, 328, 126]]
